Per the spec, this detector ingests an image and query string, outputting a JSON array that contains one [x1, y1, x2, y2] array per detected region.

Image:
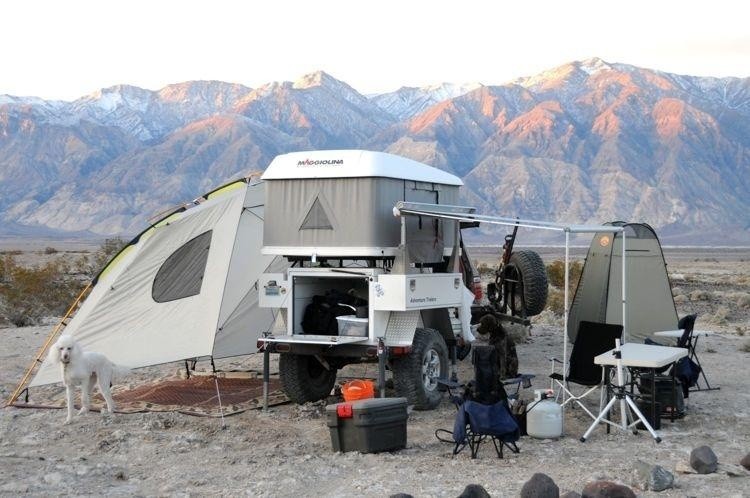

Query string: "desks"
[[581, 342, 690, 442], [427, 371, 535, 457], [652, 329, 720, 391]]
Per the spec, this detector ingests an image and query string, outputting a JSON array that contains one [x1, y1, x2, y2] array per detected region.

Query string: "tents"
[[14, 173, 284, 397], [567, 220, 682, 346]]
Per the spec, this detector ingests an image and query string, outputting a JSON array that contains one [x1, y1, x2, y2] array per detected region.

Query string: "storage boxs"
[[325, 397, 408, 453], [335, 315, 368, 336]]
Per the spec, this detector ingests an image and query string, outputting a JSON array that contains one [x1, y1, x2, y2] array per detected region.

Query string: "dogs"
[[477, 314, 519, 382], [49, 334, 134, 425]]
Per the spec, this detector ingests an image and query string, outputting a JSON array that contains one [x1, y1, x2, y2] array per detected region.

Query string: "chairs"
[[628, 313, 697, 421], [546, 321, 623, 433]]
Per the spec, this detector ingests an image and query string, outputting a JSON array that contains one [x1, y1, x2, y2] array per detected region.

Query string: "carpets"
[[11, 374, 290, 417]]
